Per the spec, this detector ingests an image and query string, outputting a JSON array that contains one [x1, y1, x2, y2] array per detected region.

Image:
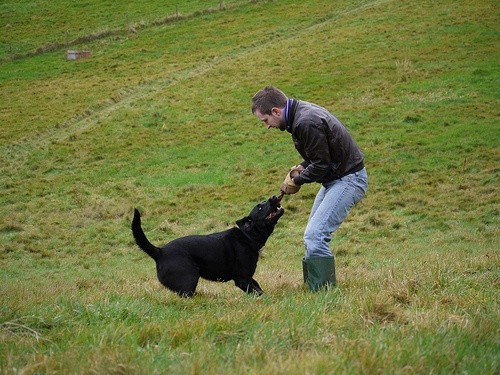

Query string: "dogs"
[[131, 194, 284, 298]]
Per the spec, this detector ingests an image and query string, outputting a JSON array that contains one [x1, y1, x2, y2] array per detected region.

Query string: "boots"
[[302, 257, 306, 285], [306, 255, 336, 293]]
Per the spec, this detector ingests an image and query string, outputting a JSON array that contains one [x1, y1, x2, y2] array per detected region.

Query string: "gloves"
[[280, 169, 300, 195]]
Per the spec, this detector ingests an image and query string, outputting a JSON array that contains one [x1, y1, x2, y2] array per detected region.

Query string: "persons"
[[252, 88, 367, 292]]
